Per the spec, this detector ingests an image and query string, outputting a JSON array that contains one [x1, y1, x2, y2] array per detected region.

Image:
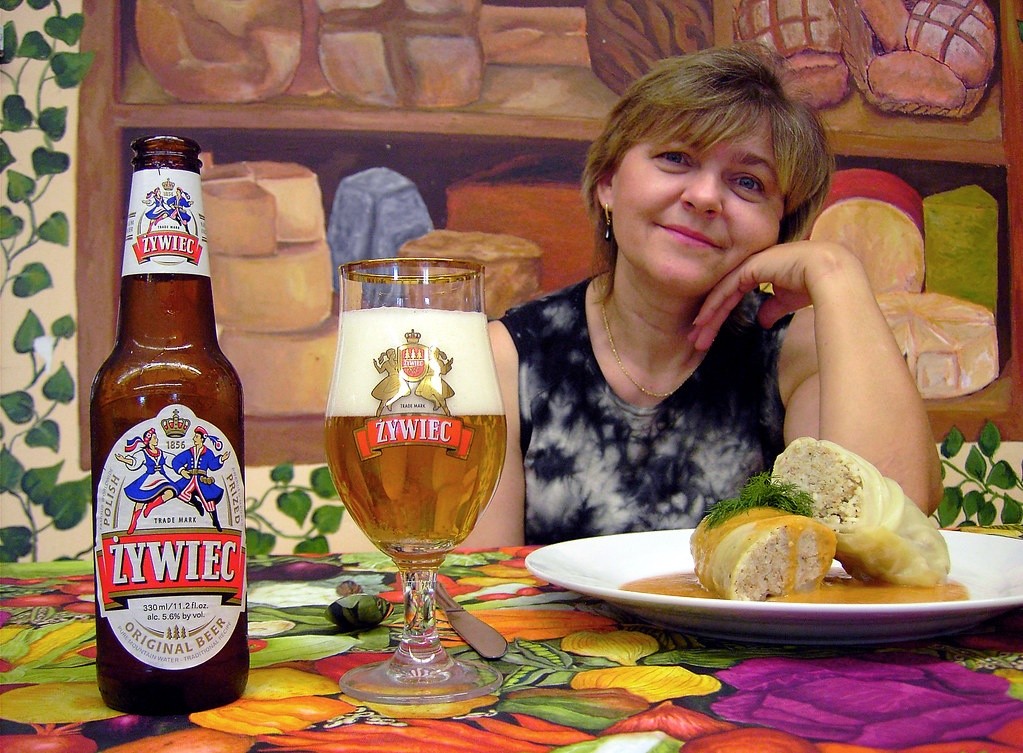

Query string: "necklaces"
[[602, 272, 714, 397]]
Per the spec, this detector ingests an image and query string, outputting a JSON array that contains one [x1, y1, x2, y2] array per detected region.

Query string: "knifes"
[[436, 582, 507, 658]]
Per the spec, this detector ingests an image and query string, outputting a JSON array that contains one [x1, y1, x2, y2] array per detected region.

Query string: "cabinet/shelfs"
[[76, 0, 1023, 475]]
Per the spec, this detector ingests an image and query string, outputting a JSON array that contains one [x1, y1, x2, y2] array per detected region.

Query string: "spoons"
[[324, 580, 394, 630]]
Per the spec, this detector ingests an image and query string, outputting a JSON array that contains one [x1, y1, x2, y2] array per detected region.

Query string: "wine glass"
[[323, 256, 507, 705]]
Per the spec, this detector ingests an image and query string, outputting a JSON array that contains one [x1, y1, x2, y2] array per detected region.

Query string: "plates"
[[524, 529, 1023, 645]]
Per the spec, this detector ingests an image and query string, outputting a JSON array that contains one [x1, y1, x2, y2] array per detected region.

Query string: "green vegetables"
[[700, 471, 817, 534]]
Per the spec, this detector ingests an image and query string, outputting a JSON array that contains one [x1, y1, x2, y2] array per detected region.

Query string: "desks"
[[0, 545, 1023, 753]]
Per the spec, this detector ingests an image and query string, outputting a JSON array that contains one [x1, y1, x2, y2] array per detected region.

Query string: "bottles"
[[90, 135, 250, 717]]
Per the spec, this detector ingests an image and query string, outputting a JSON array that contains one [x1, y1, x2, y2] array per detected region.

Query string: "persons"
[[455, 44, 944, 549]]
